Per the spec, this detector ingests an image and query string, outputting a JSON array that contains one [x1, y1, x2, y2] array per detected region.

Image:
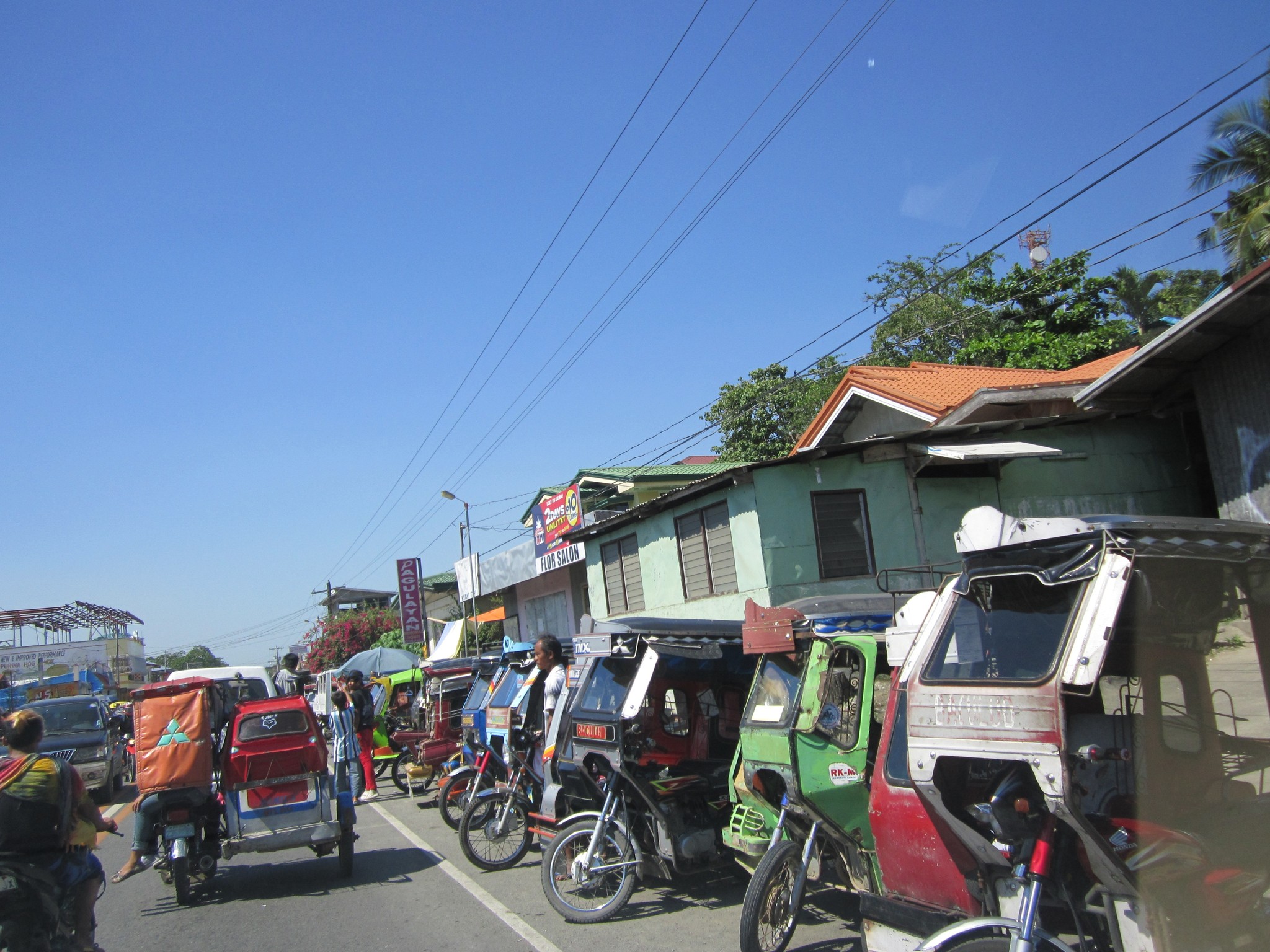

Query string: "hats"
[[346, 670, 363, 679]]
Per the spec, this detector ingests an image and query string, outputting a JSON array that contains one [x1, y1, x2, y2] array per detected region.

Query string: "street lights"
[[305, 621, 320, 648], [440, 490, 481, 656]]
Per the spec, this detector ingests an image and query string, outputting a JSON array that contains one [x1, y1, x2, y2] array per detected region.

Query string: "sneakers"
[[361, 789, 380, 800]]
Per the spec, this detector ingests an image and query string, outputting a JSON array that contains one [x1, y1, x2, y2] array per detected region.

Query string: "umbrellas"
[[333, 645, 425, 676]]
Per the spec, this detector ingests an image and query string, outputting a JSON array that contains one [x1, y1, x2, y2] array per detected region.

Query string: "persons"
[[201, 737, 221, 858], [346, 669, 378, 800], [272, 653, 304, 698], [120, 702, 135, 739], [534, 633, 565, 743], [0, 709, 118, 952], [111, 786, 209, 883], [328, 685, 362, 805]]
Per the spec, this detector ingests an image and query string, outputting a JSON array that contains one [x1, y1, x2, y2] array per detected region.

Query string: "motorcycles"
[[0, 615, 748, 952], [721, 589, 949, 952], [866, 502, 1269, 952]]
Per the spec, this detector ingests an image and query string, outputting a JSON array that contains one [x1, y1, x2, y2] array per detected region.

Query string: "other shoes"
[[111, 862, 145, 884], [78, 942, 105, 952], [141, 852, 162, 869], [353, 800, 361, 805]]
